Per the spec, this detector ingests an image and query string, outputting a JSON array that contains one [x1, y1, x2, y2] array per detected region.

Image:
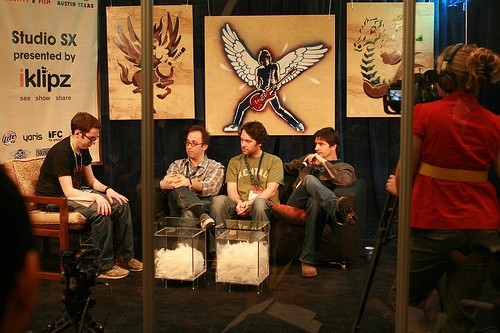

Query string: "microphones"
[[297, 157, 315, 171]]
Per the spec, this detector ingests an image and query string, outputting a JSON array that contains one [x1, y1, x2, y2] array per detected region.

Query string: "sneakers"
[[115, 258, 143, 272], [95, 264, 129, 279]]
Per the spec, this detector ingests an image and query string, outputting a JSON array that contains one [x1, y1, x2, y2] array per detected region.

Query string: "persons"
[[209, 120, 285, 268], [283, 127, 359, 277], [35, 112, 144, 279], [0, 164, 36, 333], [386, 43, 500, 333], [159, 125, 224, 250]]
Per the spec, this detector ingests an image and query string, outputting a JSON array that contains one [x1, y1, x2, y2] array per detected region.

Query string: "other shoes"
[[301, 263, 317, 277], [211, 257, 216, 270], [337, 197, 360, 226], [200, 213, 215, 230]]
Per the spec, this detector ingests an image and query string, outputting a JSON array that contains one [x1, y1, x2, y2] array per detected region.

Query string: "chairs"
[[0, 155, 88, 282]]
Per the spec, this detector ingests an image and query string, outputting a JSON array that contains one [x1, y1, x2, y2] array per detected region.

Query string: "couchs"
[[136, 160, 365, 272]]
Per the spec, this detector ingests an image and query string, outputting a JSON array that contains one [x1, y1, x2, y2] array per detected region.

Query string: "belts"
[[417, 161, 489, 183]]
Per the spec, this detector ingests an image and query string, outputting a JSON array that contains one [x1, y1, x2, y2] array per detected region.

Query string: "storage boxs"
[[152, 217, 206, 290], [214, 220, 270, 295]]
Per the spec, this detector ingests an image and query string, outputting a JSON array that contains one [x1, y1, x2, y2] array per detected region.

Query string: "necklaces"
[[70, 142, 82, 173]]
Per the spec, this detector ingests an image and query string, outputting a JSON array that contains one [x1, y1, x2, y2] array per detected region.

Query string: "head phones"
[[437, 43, 465, 94]]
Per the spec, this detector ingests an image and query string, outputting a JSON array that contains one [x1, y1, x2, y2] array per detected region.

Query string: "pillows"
[[271, 204, 306, 226]]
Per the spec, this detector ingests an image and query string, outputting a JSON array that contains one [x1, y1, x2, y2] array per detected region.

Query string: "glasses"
[[183, 139, 203, 147], [82, 133, 100, 142]]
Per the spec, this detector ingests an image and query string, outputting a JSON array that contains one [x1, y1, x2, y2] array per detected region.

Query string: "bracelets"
[[189, 178, 192, 186], [105, 187, 111, 196]]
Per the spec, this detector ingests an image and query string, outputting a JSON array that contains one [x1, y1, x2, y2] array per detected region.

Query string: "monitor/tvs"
[[389, 88, 402, 102]]
